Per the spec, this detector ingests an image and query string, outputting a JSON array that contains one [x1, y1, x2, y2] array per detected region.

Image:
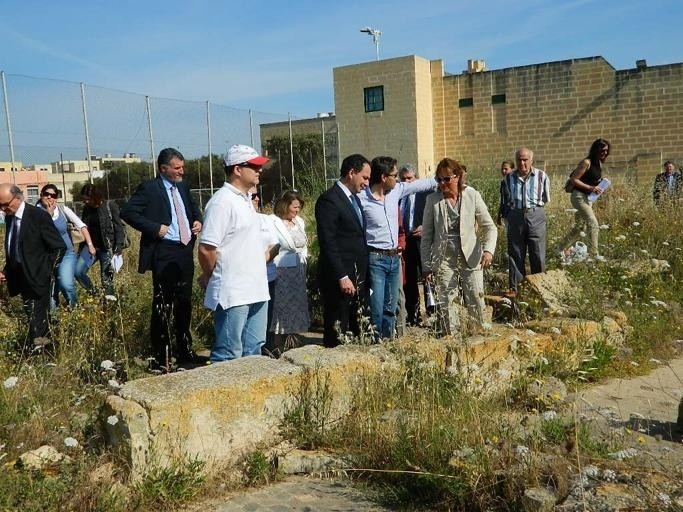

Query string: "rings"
[[489, 262, 491, 266]]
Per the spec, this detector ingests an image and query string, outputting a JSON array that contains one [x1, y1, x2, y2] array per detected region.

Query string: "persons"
[[269, 191, 310, 349], [420, 158, 497, 338], [0, 182, 67, 352], [37, 184, 96, 311], [120, 147, 203, 370], [504, 147, 550, 291], [75, 184, 123, 294], [497, 159, 515, 226], [359, 156, 438, 345], [553, 138, 611, 263], [653, 161, 683, 203], [248, 186, 281, 331], [196, 143, 270, 364], [399, 163, 436, 327], [395, 203, 406, 336], [315, 154, 372, 347]]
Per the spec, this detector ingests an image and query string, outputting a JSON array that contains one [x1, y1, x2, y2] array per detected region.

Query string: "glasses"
[[384, 173, 399, 177], [0, 193, 17, 211], [41, 190, 56, 199], [436, 175, 458, 183], [250, 192, 258, 202]]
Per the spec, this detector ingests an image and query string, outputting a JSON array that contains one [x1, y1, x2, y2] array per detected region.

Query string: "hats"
[[222, 144, 271, 165]]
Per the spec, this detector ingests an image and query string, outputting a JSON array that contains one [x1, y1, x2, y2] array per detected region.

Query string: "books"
[[589, 179, 611, 203]]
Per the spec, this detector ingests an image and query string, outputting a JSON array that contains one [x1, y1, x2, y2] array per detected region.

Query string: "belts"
[[511, 207, 540, 214], [368, 247, 402, 256]]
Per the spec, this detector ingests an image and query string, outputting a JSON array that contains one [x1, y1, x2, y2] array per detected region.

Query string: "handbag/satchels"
[[68, 214, 85, 246]]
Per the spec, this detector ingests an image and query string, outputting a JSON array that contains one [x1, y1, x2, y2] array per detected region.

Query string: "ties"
[[349, 194, 363, 227], [171, 186, 192, 246], [404, 196, 412, 233], [8, 216, 19, 272]]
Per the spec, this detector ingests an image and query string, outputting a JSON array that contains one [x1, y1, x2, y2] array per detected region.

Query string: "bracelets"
[[88, 243, 92, 245]]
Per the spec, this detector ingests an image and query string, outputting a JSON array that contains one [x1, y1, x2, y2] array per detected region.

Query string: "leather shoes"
[[180, 354, 208, 367], [152, 360, 176, 369]]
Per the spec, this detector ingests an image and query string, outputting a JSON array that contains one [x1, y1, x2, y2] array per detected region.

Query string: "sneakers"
[[504, 291, 518, 298], [586, 256, 605, 259], [553, 246, 564, 263]]
